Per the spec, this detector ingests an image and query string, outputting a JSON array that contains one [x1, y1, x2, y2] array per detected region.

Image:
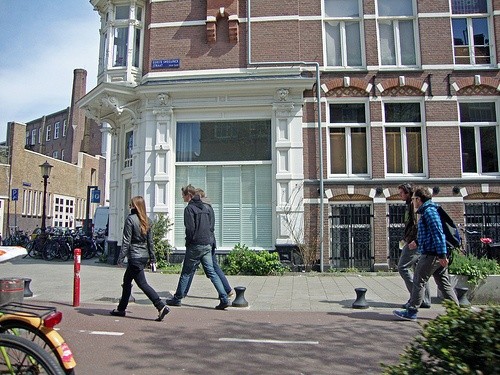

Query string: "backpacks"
[[423, 204, 461, 250]]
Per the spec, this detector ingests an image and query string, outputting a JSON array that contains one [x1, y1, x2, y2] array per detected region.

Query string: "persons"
[[392, 189, 460, 320], [397, 183, 431, 308], [166, 185, 232, 309], [185, 188, 233, 297], [109, 195, 170, 321]]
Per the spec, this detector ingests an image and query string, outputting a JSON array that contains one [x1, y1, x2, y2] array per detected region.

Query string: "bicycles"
[[0, 223, 108, 261], [0, 243, 76, 375], [0, 333, 62, 375], [455, 220, 500, 265]]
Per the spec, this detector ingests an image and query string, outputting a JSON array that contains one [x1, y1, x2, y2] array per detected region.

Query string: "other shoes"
[[166, 299, 180, 306], [216, 300, 232, 309], [110, 309, 125, 316], [421, 305, 431, 308], [228, 291, 233, 297], [156, 307, 170, 321]]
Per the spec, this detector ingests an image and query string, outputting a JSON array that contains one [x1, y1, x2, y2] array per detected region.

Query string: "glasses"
[[411, 197, 418, 201]]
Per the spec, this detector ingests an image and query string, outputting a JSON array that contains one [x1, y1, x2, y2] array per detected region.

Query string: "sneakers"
[[393, 310, 417, 321]]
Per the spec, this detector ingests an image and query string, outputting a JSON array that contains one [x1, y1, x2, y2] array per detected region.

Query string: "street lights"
[[37, 160, 54, 242]]
